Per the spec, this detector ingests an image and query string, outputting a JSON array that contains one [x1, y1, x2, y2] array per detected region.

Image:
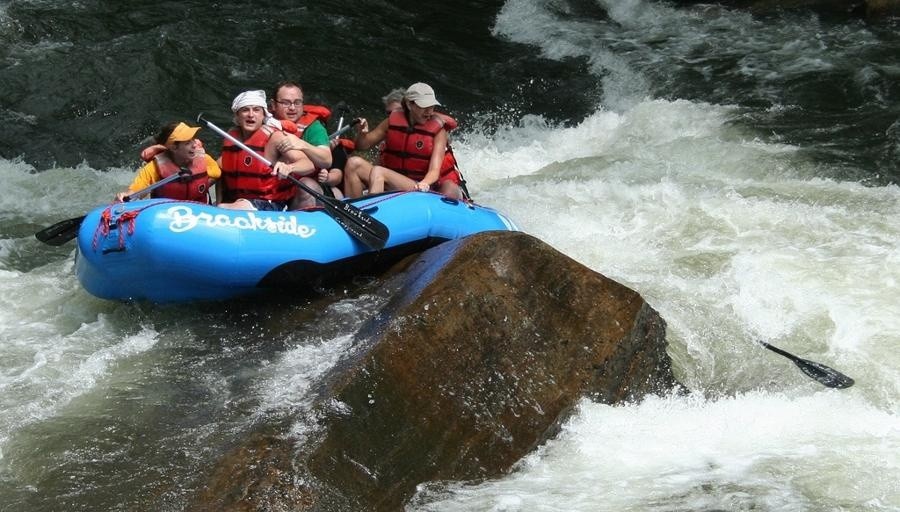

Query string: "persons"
[[353, 88, 470, 200], [269, 79, 334, 211], [344, 82, 460, 198], [316, 119, 349, 200], [113, 121, 223, 204], [208, 90, 316, 212]]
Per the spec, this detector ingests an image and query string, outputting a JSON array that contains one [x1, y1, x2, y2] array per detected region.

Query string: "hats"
[[408, 82, 441, 108], [165, 121, 202, 148]]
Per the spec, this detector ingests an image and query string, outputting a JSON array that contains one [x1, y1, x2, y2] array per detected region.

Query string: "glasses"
[[272, 97, 304, 107]]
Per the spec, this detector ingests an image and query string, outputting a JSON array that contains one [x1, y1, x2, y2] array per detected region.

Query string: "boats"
[[73, 186, 521, 302]]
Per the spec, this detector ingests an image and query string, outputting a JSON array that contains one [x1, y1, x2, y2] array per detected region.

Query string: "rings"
[[285, 143, 288, 146]]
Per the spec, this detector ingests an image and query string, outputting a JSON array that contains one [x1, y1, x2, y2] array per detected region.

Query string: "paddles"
[[759, 339, 856, 390], [34, 173, 185, 246], [194, 111, 389, 254]]
